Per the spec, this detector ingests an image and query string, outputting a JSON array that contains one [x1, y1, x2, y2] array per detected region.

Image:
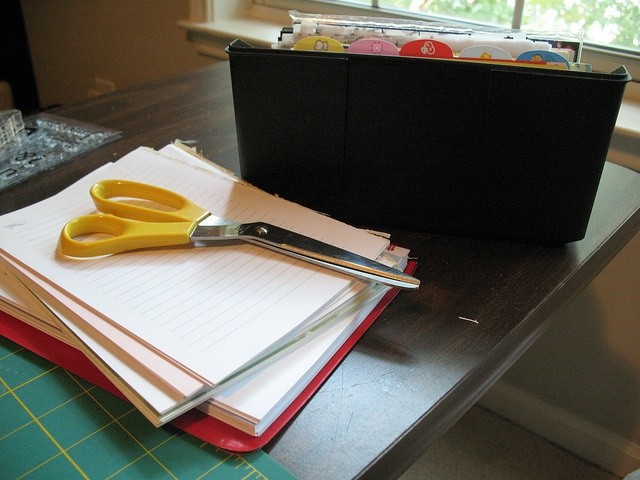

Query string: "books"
[[0, 142, 420, 456]]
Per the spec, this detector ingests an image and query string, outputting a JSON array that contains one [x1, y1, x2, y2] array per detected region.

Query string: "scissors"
[[56, 179, 420, 290]]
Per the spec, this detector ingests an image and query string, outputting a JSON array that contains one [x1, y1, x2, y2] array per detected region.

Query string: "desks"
[[0, 59, 640, 480]]
